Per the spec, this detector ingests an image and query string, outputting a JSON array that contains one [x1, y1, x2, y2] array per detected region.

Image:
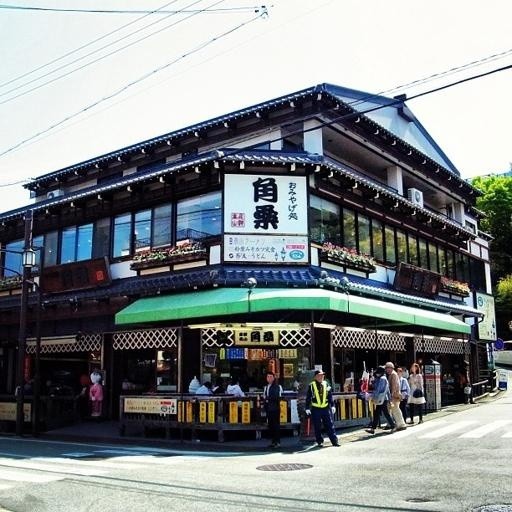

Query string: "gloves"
[[306, 410, 311, 416], [331, 407, 336, 415]]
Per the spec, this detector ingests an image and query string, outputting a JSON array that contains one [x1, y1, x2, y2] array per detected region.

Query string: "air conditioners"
[[46, 189, 65, 200], [407, 186, 424, 209]]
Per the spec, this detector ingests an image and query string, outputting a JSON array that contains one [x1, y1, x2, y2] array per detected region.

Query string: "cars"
[[491, 340, 512, 367]]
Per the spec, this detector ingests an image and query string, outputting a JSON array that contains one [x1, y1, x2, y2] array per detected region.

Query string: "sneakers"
[[333, 443, 341, 446], [318, 444, 323, 447]]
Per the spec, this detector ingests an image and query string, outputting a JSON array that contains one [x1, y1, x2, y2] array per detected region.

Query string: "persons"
[[88, 370, 104, 416], [443, 367, 476, 404], [263, 371, 281, 450], [188, 362, 427, 434], [305, 369, 341, 447]]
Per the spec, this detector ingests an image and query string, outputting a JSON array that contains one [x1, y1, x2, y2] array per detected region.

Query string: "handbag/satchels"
[[413, 390, 422, 398], [464, 386, 471, 394], [372, 393, 385, 406]]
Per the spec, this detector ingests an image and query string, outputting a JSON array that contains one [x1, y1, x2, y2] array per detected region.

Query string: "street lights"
[[17, 208, 37, 437]]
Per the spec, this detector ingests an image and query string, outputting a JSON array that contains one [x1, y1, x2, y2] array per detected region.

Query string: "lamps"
[[337, 275, 353, 295], [241, 277, 258, 294]]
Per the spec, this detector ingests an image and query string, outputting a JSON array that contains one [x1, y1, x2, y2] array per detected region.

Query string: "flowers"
[[439, 274, 472, 291], [321, 240, 376, 269], [132, 241, 205, 265]]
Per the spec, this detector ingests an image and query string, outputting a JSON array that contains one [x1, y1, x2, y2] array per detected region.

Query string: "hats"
[[384, 362, 394, 368], [315, 370, 325, 375]]
